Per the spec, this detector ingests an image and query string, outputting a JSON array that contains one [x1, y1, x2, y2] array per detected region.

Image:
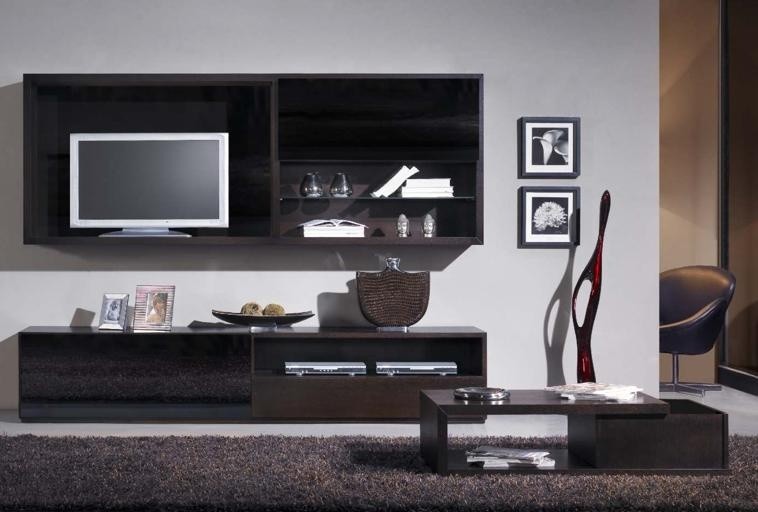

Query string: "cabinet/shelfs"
[[22, 68, 485, 245], [13, 323, 489, 424]]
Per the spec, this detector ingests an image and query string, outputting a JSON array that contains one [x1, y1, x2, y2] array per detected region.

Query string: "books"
[[465, 438, 557, 475], [370, 161, 456, 200], [297, 211, 368, 238], [548, 375, 649, 403]]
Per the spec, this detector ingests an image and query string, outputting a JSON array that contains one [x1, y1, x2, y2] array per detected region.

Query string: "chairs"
[[658, 262, 737, 395]]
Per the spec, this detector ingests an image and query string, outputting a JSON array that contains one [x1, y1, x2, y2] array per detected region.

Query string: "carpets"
[[1, 433, 756, 512]]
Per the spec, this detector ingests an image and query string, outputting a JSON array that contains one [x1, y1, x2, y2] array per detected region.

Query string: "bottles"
[[396, 214, 435, 238], [300, 172, 353, 198]]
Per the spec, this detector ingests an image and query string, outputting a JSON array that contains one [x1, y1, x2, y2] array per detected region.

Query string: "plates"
[[212, 309, 314, 326]]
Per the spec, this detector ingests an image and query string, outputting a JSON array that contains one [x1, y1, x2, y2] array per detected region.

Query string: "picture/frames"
[[519, 116, 582, 248]]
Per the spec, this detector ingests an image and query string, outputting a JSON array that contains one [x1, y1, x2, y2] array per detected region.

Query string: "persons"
[[148, 293, 169, 322], [106, 298, 120, 322]]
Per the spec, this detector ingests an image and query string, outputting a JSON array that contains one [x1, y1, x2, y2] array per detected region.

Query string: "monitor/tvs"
[[70, 132, 231, 237]]
